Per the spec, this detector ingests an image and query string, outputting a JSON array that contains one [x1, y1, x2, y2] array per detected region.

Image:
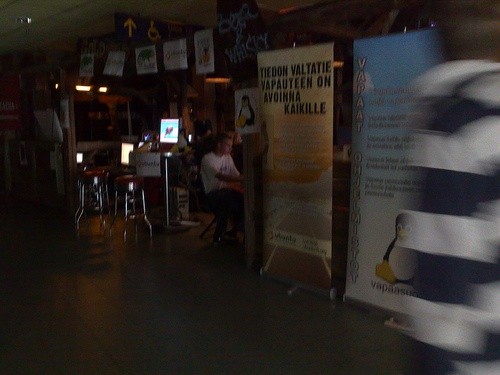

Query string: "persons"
[[199, 133, 246, 244], [397, 1, 500, 375]]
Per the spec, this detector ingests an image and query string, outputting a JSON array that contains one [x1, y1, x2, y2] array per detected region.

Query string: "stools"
[[72, 166, 110, 242], [107, 176, 155, 244]]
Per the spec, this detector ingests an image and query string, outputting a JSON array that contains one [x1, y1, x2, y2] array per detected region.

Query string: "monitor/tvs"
[[119, 142, 134, 166], [138, 142, 152, 151], [159, 118, 182, 144]]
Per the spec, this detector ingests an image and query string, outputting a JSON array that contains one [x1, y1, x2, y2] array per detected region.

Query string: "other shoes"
[[212, 233, 224, 249]]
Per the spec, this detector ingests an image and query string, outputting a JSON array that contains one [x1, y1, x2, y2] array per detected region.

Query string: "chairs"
[[188, 170, 237, 245]]
